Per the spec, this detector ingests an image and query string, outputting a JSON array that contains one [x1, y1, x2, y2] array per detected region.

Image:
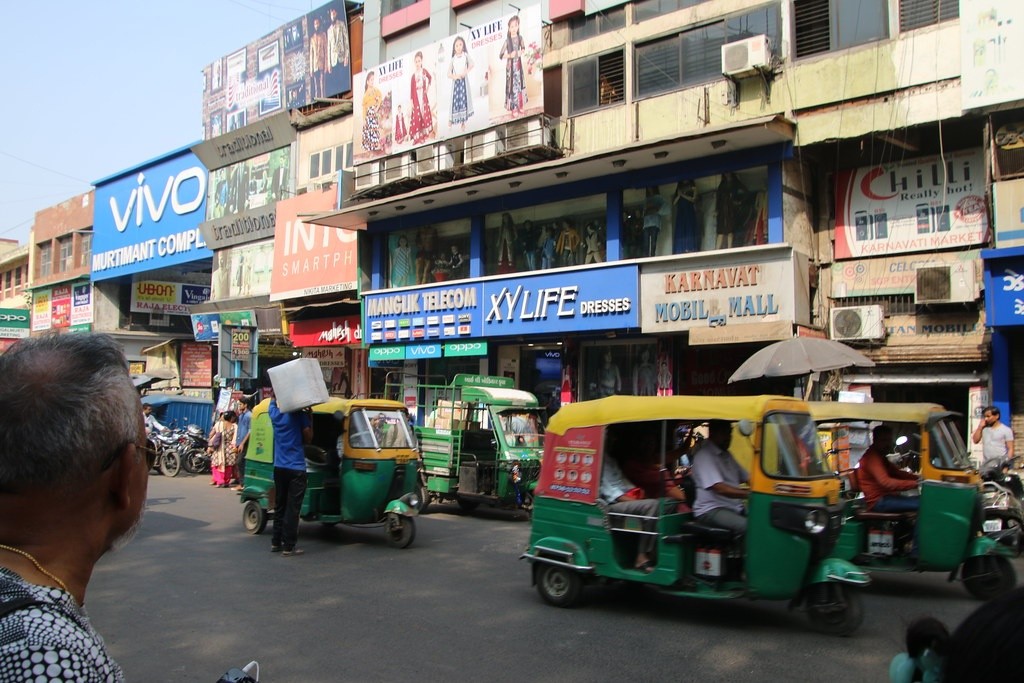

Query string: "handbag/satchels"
[[207, 421, 222, 449]]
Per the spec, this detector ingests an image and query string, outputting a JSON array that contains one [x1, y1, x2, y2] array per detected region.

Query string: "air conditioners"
[[721, 34, 771, 79], [415, 142, 455, 176], [354, 150, 415, 190], [830, 305, 887, 340], [915, 260, 977, 304], [464, 116, 551, 164]]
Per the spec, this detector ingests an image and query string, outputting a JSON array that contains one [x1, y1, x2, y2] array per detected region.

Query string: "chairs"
[[848, 469, 917, 518]]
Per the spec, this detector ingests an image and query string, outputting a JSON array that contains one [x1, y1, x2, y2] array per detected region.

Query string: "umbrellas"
[[727, 335, 876, 398]]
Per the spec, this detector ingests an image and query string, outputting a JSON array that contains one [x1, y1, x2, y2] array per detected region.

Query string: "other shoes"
[[209, 481, 217, 485], [282, 544, 305, 554], [216, 484, 229, 488], [270, 545, 282, 552]]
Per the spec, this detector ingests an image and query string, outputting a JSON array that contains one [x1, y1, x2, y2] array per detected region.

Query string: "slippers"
[[633, 559, 655, 573]]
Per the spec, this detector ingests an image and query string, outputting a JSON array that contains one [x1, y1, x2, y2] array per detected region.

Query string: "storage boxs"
[[267, 358, 329, 413]]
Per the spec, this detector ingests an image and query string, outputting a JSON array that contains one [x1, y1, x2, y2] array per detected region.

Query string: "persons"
[[448, 37, 473, 133], [428, 405, 536, 434], [673, 179, 697, 253], [499, 16, 528, 117], [207, 398, 252, 491], [410, 52, 433, 145], [371, 408, 417, 447], [599, 422, 751, 572], [642, 186, 667, 257], [143, 403, 170, 473], [392, 236, 413, 287], [310, 19, 328, 98], [363, 72, 383, 157], [0, 332, 156, 683], [415, 224, 463, 283], [713, 170, 753, 250], [858, 425, 920, 561], [268, 388, 313, 556], [396, 105, 407, 143], [974, 406, 1014, 472], [494, 212, 602, 270], [327, 8, 349, 89]]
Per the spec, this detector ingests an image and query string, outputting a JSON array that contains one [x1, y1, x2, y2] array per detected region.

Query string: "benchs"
[[461, 430, 496, 467], [538, 492, 623, 530]]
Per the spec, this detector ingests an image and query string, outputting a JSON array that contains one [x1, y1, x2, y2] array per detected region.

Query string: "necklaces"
[[0, 544, 68, 590]]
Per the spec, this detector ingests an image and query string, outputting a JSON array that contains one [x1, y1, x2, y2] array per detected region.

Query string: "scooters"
[[977, 453, 1024, 558]]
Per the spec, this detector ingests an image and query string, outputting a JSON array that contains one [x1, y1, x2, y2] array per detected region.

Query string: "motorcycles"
[[727, 402, 1024, 602], [520, 395, 873, 635], [145, 416, 212, 476], [384, 370, 546, 524], [237, 396, 422, 549]]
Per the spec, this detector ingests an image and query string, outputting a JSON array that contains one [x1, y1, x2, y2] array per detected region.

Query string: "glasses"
[[102, 436, 157, 472]]
